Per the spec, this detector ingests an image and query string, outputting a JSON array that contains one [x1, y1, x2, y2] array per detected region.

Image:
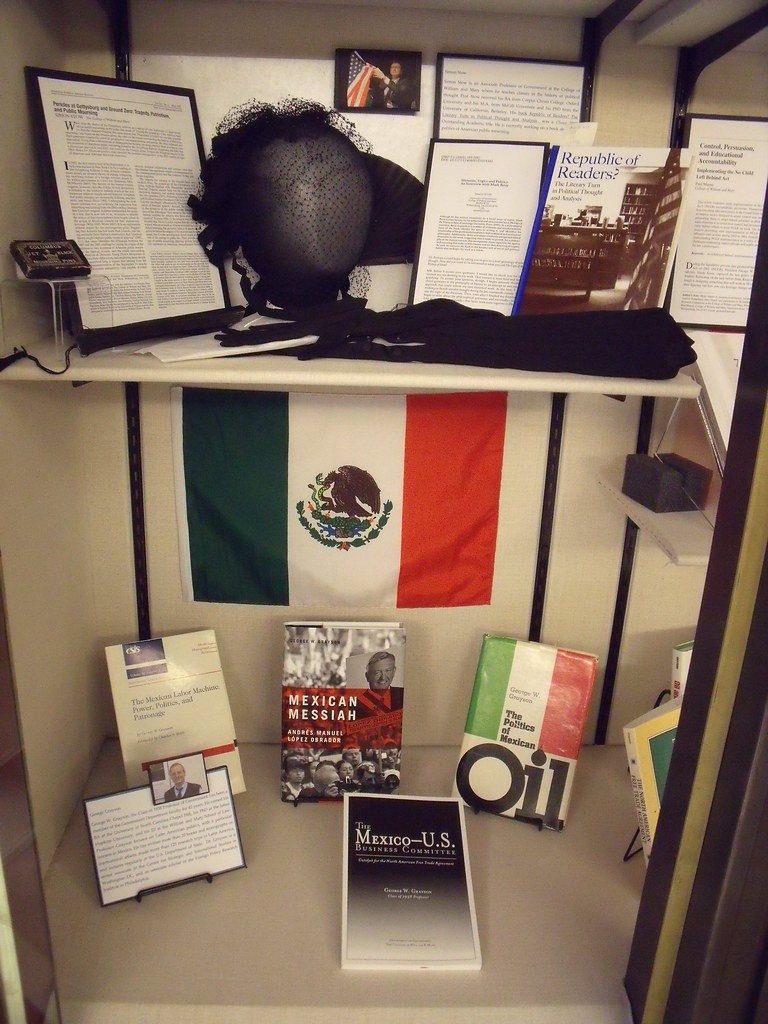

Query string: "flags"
[[346, 53, 375, 107], [171, 385, 509, 608]]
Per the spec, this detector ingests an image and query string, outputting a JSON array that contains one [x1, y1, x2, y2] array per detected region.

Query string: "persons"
[[283, 627, 404, 801], [369, 61, 413, 110], [165, 763, 202, 803], [357, 652, 404, 720]]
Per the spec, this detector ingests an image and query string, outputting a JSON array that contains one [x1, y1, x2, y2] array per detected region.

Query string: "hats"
[[342, 745, 359, 759]]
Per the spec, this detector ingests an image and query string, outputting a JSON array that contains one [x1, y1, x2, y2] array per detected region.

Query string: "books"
[[105, 629, 246, 795], [511, 145, 696, 317], [623, 696, 683, 868], [281, 622, 405, 802], [451, 632, 600, 831], [342, 792, 482, 969], [671, 639, 694, 700]]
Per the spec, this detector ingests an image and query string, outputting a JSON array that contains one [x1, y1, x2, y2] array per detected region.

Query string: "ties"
[[385, 81, 394, 101], [177, 788, 183, 798]]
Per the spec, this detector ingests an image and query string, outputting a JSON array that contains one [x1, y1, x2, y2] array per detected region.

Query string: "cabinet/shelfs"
[[0, 0, 768, 1024]]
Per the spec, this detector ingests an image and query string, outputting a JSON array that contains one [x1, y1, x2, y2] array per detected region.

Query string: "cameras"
[[332, 776, 358, 796]]
